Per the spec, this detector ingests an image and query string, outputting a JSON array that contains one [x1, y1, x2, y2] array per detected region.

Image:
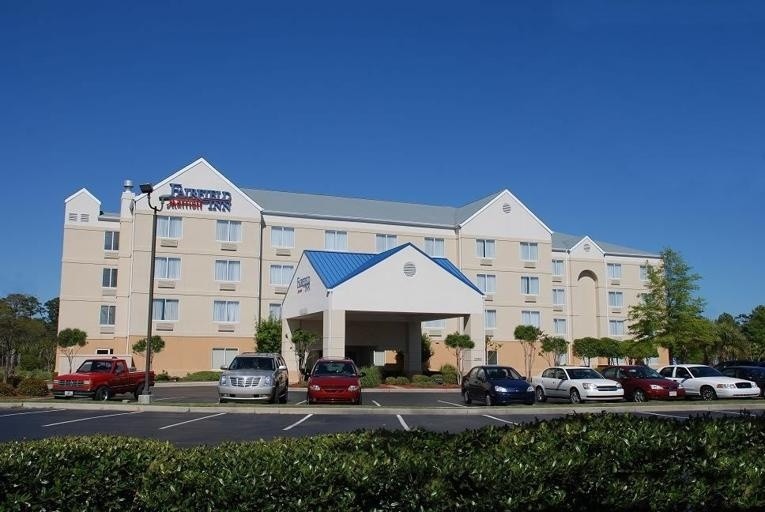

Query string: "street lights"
[[138, 182, 172, 403]]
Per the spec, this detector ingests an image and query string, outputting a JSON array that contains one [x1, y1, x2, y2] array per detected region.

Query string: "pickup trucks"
[[53, 356, 156, 400]]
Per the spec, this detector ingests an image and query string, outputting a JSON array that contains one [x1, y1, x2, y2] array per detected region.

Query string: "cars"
[[218, 352, 289, 404], [304, 357, 366, 406]]
